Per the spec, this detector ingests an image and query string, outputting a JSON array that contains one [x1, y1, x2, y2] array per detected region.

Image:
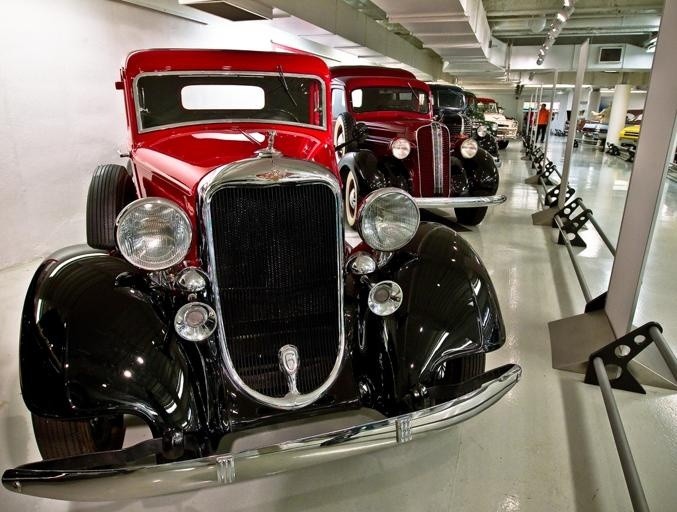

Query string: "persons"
[[534, 104, 548, 143], [522, 107, 534, 134]]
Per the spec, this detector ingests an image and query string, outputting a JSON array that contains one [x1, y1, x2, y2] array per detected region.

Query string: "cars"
[[323, 64, 502, 230], [380, 80, 522, 166], [0, 48, 521, 511], [550, 113, 641, 162]]
